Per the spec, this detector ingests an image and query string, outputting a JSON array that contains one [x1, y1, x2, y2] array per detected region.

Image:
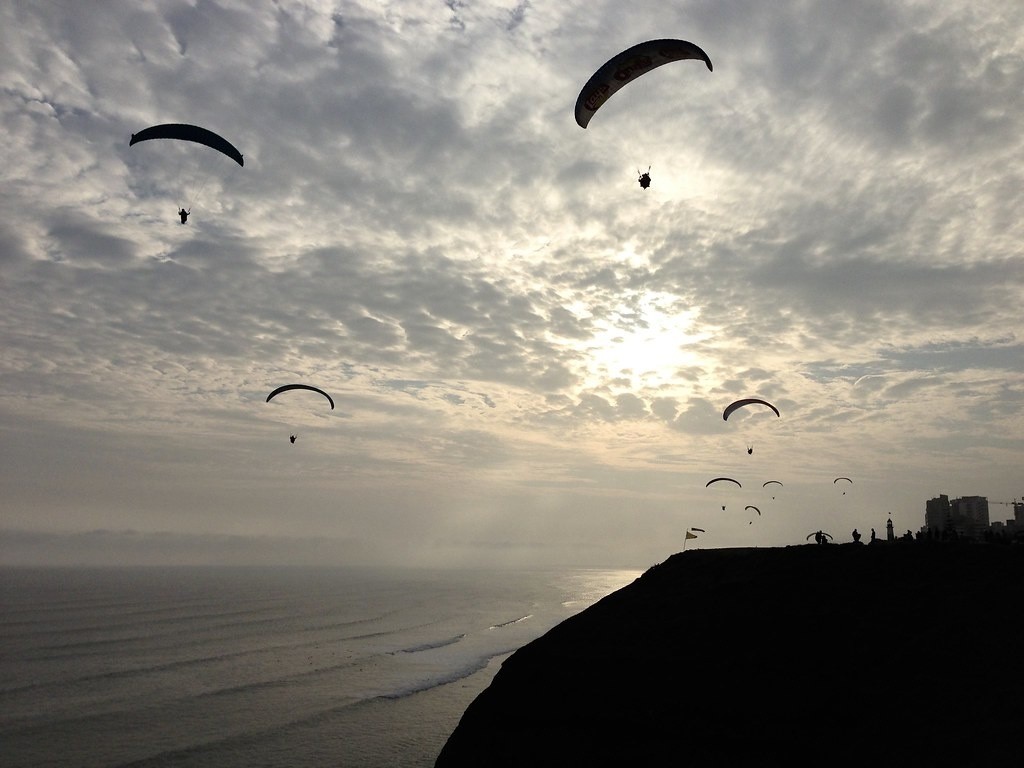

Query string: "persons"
[[815, 531, 828, 544], [852, 529, 861, 541], [178, 209, 190, 224], [638, 173, 651, 189], [871, 528, 875, 540]]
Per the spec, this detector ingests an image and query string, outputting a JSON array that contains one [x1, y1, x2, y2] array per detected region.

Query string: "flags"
[[686, 532, 697, 539]]
